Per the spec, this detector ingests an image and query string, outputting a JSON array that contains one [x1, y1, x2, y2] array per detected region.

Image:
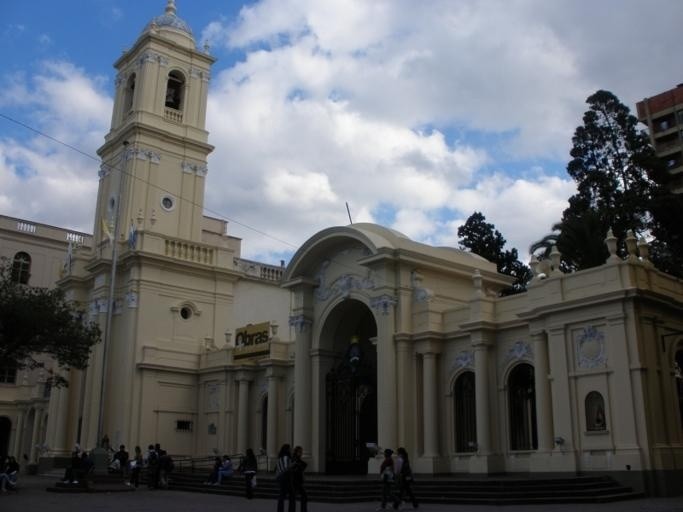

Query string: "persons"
[[211, 453, 235, 487], [392, 447, 419, 511], [238, 448, 257, 500], [201, 455, 223, 485], [375, 448, 405, 511], [274, 442, 293, 512], [57, 431, 174, 491], [2, 455, 19, 490], [290, 444, 308, 512]]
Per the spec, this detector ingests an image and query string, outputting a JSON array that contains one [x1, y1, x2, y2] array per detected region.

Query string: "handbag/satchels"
[[251, 475, 258, 489]]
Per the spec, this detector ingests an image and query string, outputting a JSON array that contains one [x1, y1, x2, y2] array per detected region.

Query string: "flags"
[[100, 220, 113, 251], [59, 243, 70, 279], [127, 222, 135, 251]]
